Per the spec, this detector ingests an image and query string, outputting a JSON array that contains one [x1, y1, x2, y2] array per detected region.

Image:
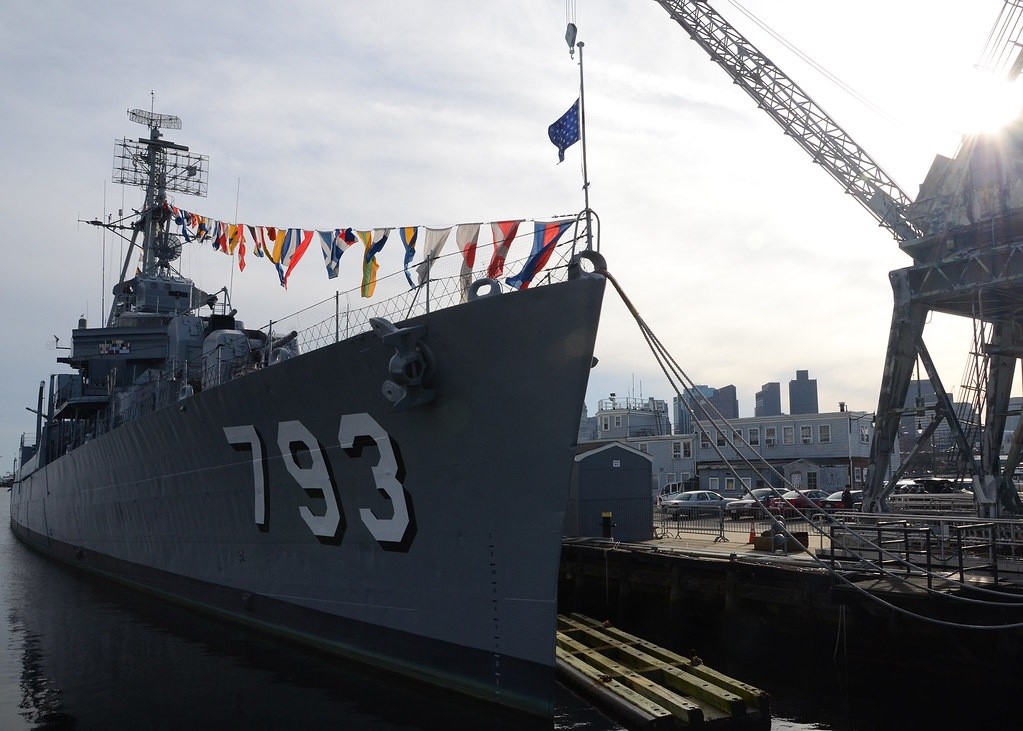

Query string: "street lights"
[[848, 411, 876, 490], [889, 419, 922, 481]]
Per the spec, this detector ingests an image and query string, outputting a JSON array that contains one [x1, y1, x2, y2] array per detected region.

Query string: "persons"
[[842, 485, 853, 520], [892, 486, 930, 501], [944, 480, 963, 493]]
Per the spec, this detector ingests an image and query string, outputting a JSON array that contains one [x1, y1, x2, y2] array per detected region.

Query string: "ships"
[[7, 41, 606, 731]]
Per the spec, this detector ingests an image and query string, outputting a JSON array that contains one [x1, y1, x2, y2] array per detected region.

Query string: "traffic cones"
[[748, 523, 757, 545]]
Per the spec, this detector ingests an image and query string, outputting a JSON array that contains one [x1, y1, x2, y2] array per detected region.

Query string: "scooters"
[[659, 482, 684, 507]]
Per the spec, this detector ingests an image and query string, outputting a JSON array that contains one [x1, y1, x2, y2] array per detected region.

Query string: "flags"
[[135, 253, 142, 275], [247, 226, 314, 289], [487, 221, 520, 281], [547, 97, 580, 165], [163, 203, 246, 272], [505, 218, 575, 290], [416, 227, 453, 289], [317, 228, 358, 279], [399, 227, 418, 290], [456, 223, 483, 292], [356, 228, 392, 298]]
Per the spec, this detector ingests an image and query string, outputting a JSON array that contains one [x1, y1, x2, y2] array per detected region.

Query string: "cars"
[[819, 490, 863, 520], [770, 489, 830, 520], [666, 491, 739, 520], [726, 488, 790, 520]]
